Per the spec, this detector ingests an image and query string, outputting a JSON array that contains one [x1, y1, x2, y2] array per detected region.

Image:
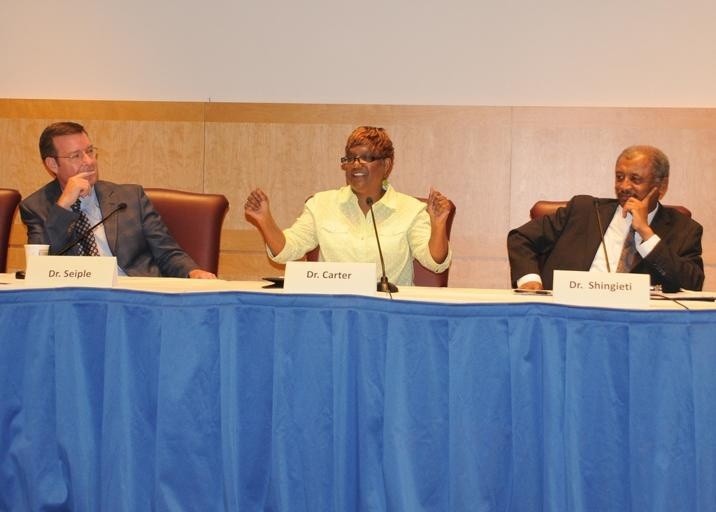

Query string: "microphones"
[[594, 197, 610, 272], [366, 196, 399, 293], [15, 202, 127, 278]]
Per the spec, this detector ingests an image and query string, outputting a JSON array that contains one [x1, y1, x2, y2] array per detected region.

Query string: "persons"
[[504, 144, 706, 295], [16, 121, 220, 281], [242, 126, 455, 285]]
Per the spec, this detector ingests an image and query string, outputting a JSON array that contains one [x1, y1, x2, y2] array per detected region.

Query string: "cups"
[[24, 244, 50, 274]]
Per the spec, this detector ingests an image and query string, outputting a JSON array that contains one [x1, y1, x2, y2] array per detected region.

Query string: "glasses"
[[340, 155, 385, 163], [53, 147, 100, 161]]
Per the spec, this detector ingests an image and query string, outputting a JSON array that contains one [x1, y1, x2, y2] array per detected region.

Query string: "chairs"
[[530, 201, 692, 224], [144, 188, 229, 277], [0, 187, 22, 273], [304, 195, 456, 288]]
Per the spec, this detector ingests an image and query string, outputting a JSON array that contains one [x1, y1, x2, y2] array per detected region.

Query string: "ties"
[[617, 223, 637, 274], [70, 197, 102, 257]]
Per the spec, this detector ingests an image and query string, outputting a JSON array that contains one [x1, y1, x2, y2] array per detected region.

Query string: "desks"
[[0, 274, 716, 512]]
